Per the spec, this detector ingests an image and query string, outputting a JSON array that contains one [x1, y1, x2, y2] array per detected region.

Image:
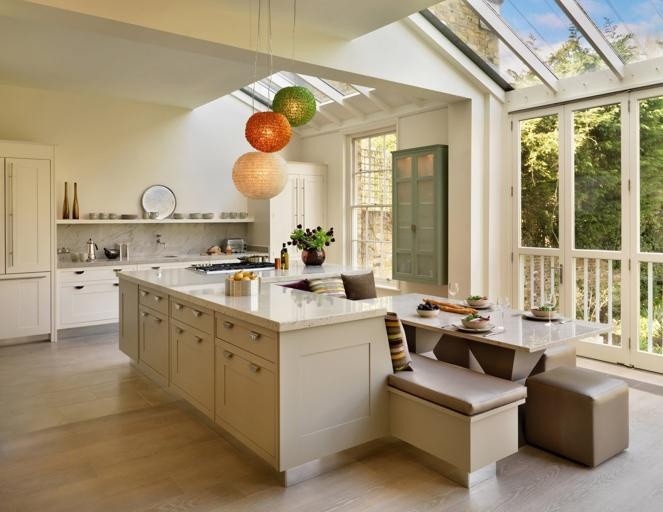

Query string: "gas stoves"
[[185, 263, 274, 274]]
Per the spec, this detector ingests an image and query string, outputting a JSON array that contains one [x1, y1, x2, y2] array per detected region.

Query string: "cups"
[[78, 252, 87, 262], [69, 253, 79, 262], [172, 212, 246, 219], [87, 213, 118, 220], [275, 258, 280, 269]]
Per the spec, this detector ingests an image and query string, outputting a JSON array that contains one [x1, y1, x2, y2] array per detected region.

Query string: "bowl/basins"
[[464, 299, 488, 306], [461, 318, 489, 328], [530, 307, 558, 317], [142, 211, 159, 219], [415, 306, 439, 317], [223, 279, 260, 297]]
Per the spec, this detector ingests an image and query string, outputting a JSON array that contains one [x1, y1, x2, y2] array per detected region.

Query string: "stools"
[[526, 365, 630, 467]]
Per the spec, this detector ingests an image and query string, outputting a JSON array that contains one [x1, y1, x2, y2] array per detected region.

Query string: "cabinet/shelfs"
[[57, 267, 119, 330], [1, 139, 63, 345], [247, 163, 327, 264], [115, 258, 392, 485], [390, 144, 448, 285]]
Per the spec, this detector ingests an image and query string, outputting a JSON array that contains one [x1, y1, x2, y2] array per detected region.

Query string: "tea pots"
[[85, 238, 97, 261]]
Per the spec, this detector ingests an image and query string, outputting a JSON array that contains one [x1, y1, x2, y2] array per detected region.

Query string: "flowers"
[[287, 224, 337, 252]]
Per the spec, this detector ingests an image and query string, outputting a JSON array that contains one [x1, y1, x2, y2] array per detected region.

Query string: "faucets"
[[160, 242, 166, 248]]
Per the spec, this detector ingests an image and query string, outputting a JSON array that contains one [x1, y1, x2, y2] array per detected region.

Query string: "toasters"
[[222, 238, 247, 253]]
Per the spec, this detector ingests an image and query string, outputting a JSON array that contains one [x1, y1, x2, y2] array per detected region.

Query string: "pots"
[[102, 247, 123, 259], [237, 254, 268, 264]]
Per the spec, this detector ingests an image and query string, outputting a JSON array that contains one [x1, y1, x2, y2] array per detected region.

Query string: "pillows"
[[382, 312, 415, 371], [284, 271, 376, 300]]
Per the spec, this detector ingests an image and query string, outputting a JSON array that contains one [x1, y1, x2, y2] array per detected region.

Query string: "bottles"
[[280, 243, 289, 270], [62, 182, 69, 219], [71, 182, 79, 219], [226, 245, 231, 255]]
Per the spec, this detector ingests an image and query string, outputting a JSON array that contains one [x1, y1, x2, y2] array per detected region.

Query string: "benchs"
[[358, 362, 528, 488]]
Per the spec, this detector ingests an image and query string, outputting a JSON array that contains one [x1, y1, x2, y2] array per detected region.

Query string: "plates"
[[199, 252, 217, 256], [522, 311, 561, 320], [462, 304, 494, 309], [121, 214, 137, 219], [455, 324, 494, 333]]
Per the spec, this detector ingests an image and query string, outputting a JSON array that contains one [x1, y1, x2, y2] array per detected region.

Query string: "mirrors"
[[140, 186, 177, 219]]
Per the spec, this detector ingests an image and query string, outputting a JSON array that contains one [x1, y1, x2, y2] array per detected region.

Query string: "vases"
[[301, 248, 326, 266]]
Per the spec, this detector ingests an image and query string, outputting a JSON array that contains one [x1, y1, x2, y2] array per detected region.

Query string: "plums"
[[229, 271, 255, 281]]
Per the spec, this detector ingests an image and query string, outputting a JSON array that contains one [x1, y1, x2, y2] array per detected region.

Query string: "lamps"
[[232, 0, 317, 198]]
